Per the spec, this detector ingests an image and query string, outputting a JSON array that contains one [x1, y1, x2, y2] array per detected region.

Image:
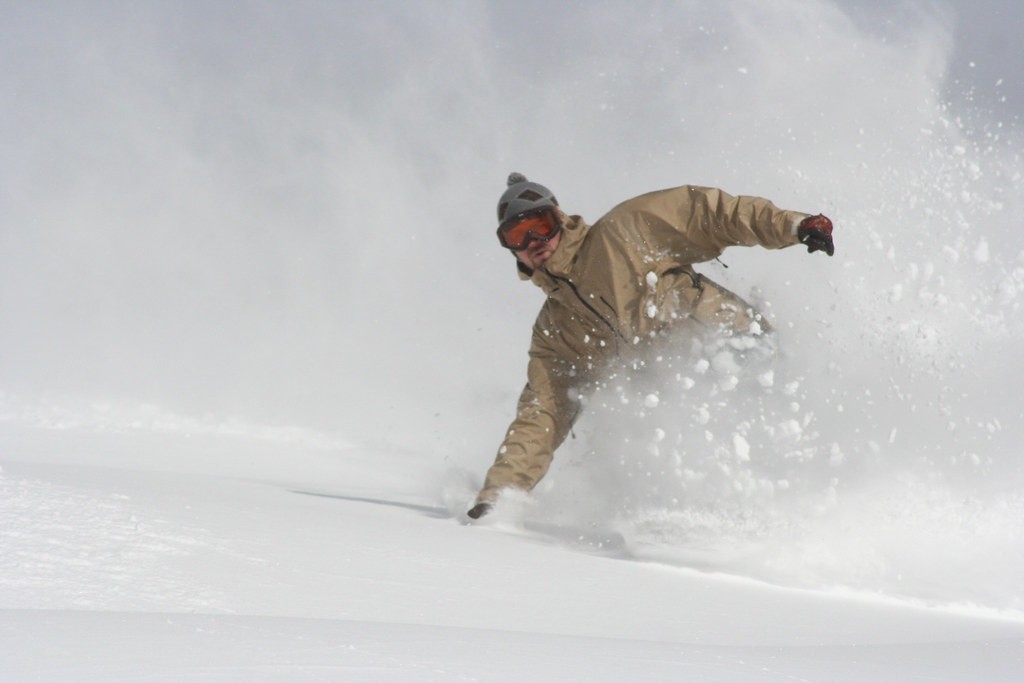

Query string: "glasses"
[[496, 205, 561, 251]]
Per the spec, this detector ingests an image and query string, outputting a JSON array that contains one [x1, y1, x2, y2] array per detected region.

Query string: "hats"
[[497, 172, 560, 225]]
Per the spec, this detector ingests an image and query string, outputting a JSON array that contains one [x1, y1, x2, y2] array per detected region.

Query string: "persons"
[[466, 172, 836, 522]]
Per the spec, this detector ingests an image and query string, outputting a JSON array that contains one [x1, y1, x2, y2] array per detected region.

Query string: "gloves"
[[468, 503, 489, 520], [797, 213, 836, 257]]
